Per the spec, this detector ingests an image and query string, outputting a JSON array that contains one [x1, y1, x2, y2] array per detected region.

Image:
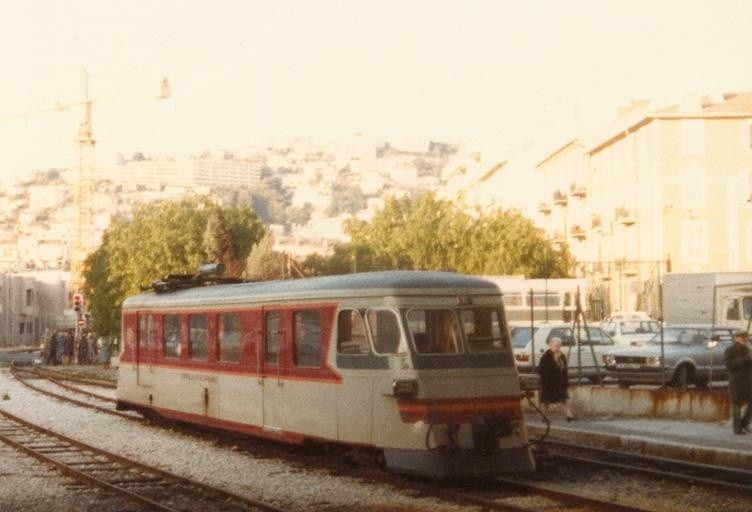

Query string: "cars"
[[501, 305, 747, 391]]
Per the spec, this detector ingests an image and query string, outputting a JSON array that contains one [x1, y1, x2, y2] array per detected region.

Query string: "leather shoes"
[[566, 415, 578, 422], [541, 418, 551, 423]]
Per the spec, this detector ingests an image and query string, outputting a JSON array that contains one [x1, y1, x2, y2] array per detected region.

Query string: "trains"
[[106, 260, 550, 493]]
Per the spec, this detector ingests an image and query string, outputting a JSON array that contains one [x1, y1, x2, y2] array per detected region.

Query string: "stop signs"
[[77, 319, 85, 327]]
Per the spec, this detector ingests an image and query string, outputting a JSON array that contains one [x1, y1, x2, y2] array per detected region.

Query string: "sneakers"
[[735, 430, 745, 434], [741, 420, 750, 431]]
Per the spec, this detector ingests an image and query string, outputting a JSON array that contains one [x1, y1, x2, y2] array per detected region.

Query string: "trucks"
[[656, 268, 752, 335]]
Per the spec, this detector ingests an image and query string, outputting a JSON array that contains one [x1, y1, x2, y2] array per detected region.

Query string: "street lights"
[[533, 176, 639, 311]]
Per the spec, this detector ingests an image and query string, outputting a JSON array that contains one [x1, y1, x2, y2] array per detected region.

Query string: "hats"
[[734, 331, 747, 337]]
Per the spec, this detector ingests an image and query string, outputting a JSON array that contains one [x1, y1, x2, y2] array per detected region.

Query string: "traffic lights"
[[73, 292, 84, 314]]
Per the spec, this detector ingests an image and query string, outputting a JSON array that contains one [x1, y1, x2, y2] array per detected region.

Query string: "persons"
[[536, 335, 579, 422], [721, 326, 751, 435], [36, 327, 113, 371]]
